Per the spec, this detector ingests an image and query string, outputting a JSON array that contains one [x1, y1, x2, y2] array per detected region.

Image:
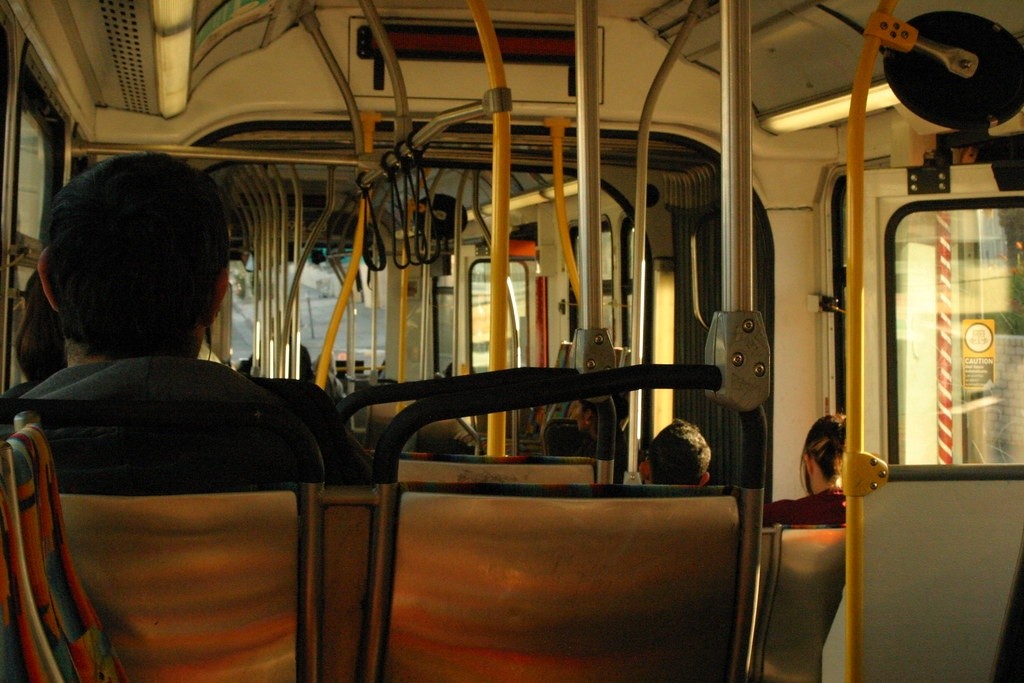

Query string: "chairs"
[[1, 365, 846, 683]]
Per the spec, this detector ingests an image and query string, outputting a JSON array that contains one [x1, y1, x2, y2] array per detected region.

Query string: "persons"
[[0, 151, 372, 499], [764, 414, 847, 524], [639, 422, 711, 486], [559, 401, 629, 483]]
